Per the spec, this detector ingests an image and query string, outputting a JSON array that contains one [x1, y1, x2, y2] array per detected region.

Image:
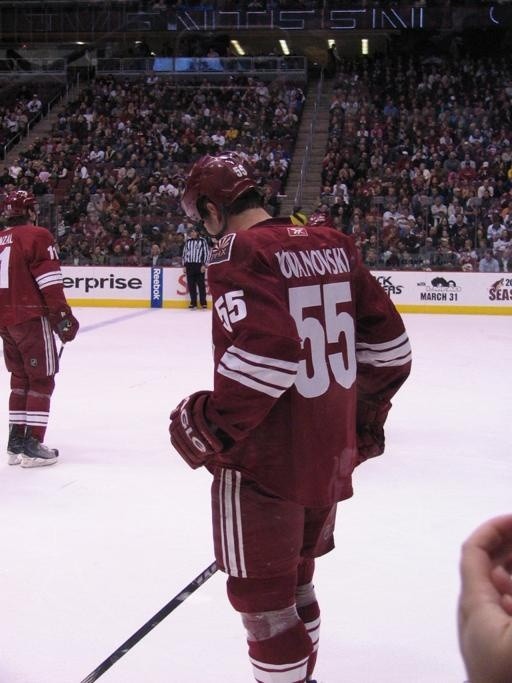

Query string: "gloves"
[[169, 390, 223, 468], [357, 431, 385, 464], [53, 305, 79, 344]]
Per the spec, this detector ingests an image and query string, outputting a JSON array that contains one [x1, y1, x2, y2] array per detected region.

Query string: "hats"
[[483, 161, 489, 167]]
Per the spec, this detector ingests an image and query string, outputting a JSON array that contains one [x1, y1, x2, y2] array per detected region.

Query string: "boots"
[[7, 436, 59, 458]]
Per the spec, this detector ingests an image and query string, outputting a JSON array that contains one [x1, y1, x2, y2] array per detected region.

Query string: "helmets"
[[2, 191, 34, 217], [180, 151, 256, 221]]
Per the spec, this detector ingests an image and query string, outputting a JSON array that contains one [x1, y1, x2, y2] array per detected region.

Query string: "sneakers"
[[189, 305, 207, 308]]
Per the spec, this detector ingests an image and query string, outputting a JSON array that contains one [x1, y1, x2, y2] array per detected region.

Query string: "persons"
[[1, 45, 305, 266], [454, 513, 511, 683], [1, 185, 80, 462], [181, 225, 211, 310], [310, 44, 512, 273], [168, 149, 416, 683]]
